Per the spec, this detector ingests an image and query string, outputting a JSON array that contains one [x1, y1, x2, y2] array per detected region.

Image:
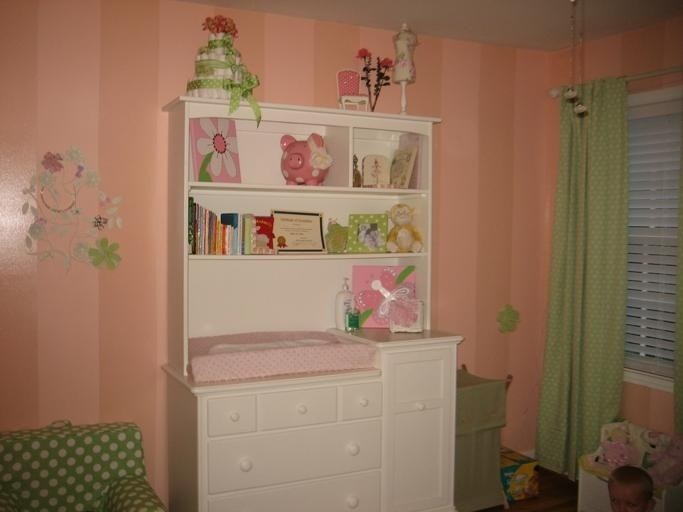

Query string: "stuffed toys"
[[384, 203, 425, 253]]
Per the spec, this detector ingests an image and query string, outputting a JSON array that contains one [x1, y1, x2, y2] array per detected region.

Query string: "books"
[[390, 145, 418, 189], [188, 118, 243, 185], [186, 196, 277, 255]]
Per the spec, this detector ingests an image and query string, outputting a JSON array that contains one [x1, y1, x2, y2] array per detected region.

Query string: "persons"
[[363, 228, 376, 249], [608, 465, 657, 511], [358, 224, 367, 241], [390, 22, 420, 85]]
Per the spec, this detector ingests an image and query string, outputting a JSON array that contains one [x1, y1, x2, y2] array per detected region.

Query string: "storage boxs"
[[501, 446, 539, 502]]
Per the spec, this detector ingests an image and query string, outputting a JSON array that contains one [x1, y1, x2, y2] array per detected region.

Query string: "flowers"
[[356, 47, 393, 113]]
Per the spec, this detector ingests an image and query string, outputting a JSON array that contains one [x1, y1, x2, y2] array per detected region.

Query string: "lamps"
[[392, 22, 418, 114]]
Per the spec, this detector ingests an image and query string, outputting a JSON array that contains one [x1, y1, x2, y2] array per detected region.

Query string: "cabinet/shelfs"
[[160, 370, 382, 512], [454, 364, 512, 512], [375, 335, 458, 512], [161, 96, 441, 378]]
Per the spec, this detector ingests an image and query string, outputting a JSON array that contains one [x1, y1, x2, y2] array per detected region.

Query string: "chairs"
[[0, 418, 168, 512], [336, 69, 366, 114]]
